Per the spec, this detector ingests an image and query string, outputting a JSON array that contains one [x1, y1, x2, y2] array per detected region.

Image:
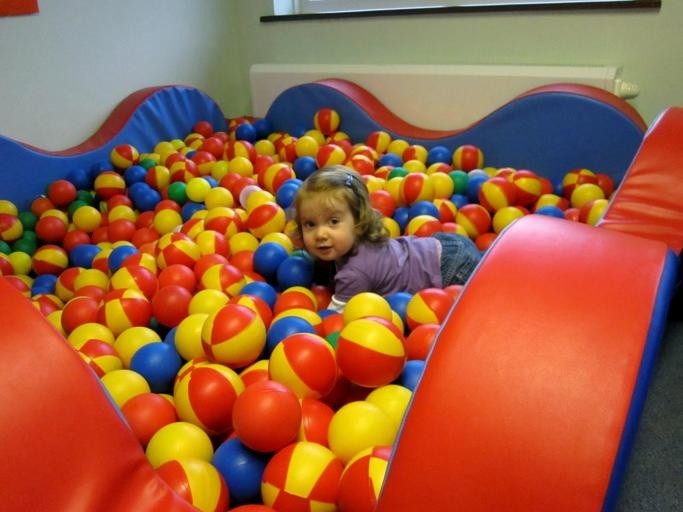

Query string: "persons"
[[291, 165, 482, 312]]
